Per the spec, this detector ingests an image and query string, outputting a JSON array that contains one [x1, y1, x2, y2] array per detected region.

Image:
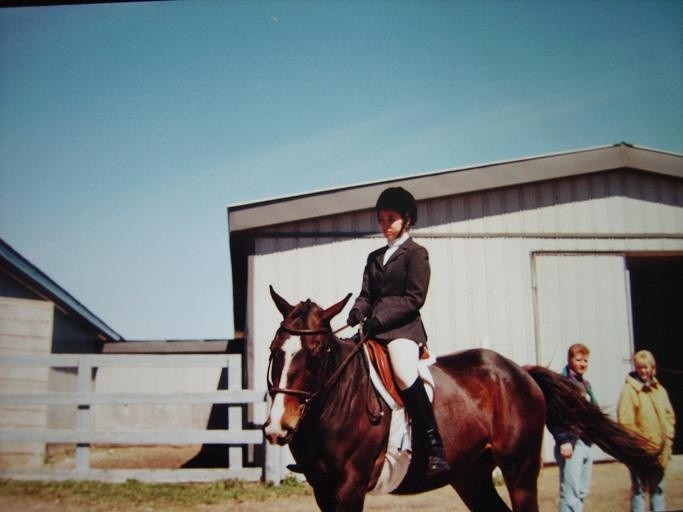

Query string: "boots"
[[424, 429, 450, 474]]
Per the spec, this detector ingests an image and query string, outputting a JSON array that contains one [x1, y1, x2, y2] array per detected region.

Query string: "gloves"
[[347, 307, 360, 325], [363, 316, 382, 332]]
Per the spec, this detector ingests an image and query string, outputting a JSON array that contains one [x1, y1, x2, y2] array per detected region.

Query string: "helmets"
[[375, 186, 417, 225]]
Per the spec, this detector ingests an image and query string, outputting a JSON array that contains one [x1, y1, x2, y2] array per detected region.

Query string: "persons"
[[285, 183, 451, 482], [551, 341, 598, 511], [616, 348, 677, 512]]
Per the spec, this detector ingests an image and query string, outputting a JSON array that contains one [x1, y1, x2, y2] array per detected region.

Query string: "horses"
[[264, 284, 668, 512]]
